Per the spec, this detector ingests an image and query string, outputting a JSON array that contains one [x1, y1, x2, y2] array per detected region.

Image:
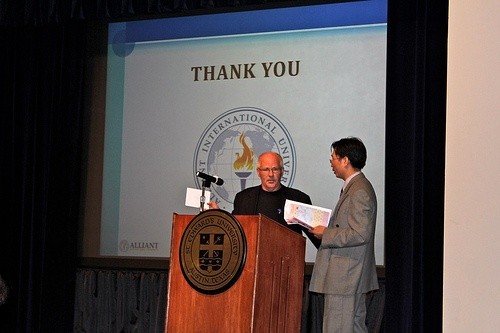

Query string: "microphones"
[[195, 171, 224, 186]]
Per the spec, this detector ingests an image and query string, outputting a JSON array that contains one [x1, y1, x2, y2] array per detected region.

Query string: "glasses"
[[257, 166, 282, 172], [330, 155, 345, 164]]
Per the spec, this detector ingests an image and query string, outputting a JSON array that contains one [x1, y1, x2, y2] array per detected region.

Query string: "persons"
[[206, 151, 322, 250], [309, 137, 379, 333]]
